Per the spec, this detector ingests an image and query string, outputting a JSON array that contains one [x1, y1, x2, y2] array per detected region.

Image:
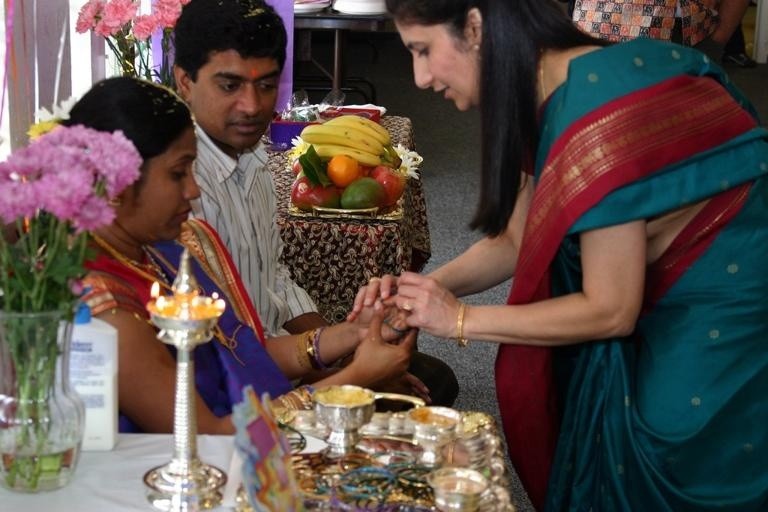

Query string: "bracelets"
[[456, 302, 467, 347], [277, 420, 435, 511], [296, 322, 339, 374], [375, 294, 414, 334]]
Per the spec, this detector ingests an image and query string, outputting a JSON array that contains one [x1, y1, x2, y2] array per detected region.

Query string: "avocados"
[[340, 176, 386, 210]]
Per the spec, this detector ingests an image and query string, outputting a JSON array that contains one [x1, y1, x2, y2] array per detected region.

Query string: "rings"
[[404, 301, 412, 313]]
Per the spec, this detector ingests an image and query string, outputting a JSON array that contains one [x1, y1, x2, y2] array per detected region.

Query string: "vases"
[[0, 311, 85, 492]]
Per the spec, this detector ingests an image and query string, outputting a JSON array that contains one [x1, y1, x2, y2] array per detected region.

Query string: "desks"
[[264, 113, 431, 324], [294, 11, 382, 109], [0, 432, 234, 511]]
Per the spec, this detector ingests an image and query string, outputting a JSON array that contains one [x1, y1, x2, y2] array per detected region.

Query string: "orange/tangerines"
[[327, 155, 358, 189]]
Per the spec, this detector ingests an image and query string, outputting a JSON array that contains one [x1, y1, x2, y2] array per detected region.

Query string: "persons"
[[347, 0, 768, 511], [171, 1, 461, 407], [53, 75, 419, 434]]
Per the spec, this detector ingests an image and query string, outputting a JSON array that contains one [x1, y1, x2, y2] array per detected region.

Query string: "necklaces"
[[538, 48, 546, 101]]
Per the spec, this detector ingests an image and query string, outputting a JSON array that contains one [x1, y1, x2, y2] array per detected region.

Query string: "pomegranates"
[[291, 176, 340, 212], [370, 166, 407, 207]]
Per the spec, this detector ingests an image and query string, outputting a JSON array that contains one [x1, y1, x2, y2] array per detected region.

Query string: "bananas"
[[300, 114, 402, 171]]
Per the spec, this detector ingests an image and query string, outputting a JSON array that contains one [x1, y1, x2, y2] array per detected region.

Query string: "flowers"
[[75, 1, 185, 89], [0, 126, 140, 489]]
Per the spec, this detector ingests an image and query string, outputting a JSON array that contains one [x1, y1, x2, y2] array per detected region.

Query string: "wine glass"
[[315, 384, 490, 511]]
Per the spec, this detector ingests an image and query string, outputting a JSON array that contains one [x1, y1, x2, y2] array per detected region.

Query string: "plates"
[[286, 176, 405, 221], [294, 0, 387, 17]]
